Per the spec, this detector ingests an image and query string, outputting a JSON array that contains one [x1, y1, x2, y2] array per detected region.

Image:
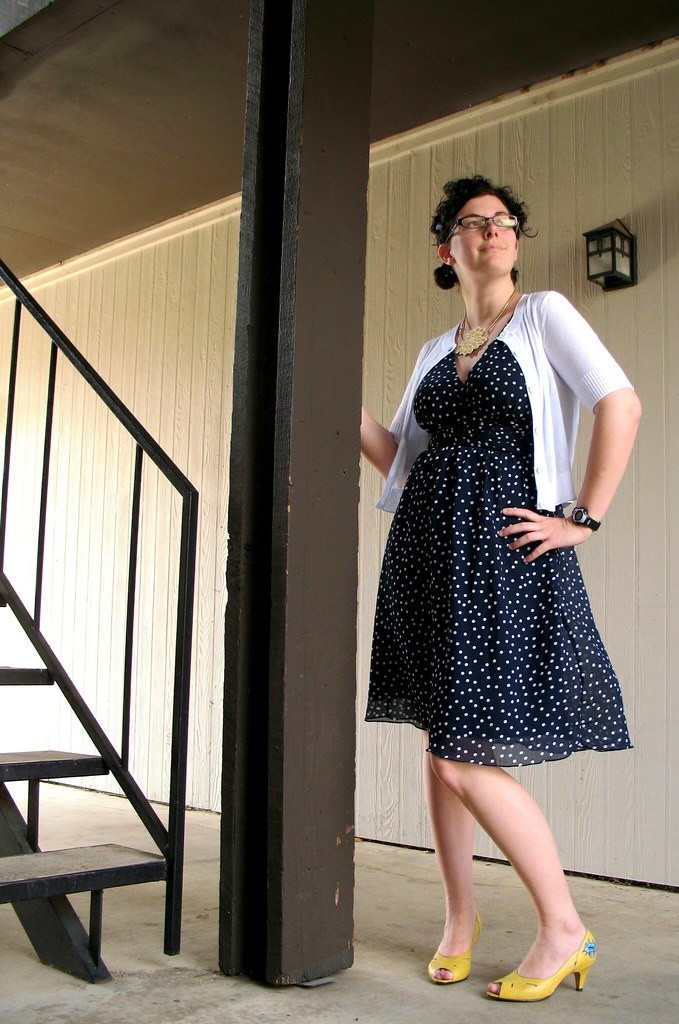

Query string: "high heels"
[[428, 911, 481, 985], [485, 928, 597, 1001]]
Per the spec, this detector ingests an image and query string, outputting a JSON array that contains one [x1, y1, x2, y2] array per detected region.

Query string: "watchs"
[[571, 507, 601, 531]]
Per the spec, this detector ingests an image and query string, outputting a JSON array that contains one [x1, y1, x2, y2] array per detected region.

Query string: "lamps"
[[583, 217, 637, 293]]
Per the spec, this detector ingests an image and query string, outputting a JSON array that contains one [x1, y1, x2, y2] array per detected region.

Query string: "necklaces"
[[454, 290, 517, 356]]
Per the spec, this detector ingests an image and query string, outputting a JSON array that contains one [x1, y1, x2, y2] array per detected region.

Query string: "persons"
[[360, 175, 641, 1002]]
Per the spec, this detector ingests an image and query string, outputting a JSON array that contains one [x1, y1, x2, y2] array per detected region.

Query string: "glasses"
[[445, 215, 519, 245]]
